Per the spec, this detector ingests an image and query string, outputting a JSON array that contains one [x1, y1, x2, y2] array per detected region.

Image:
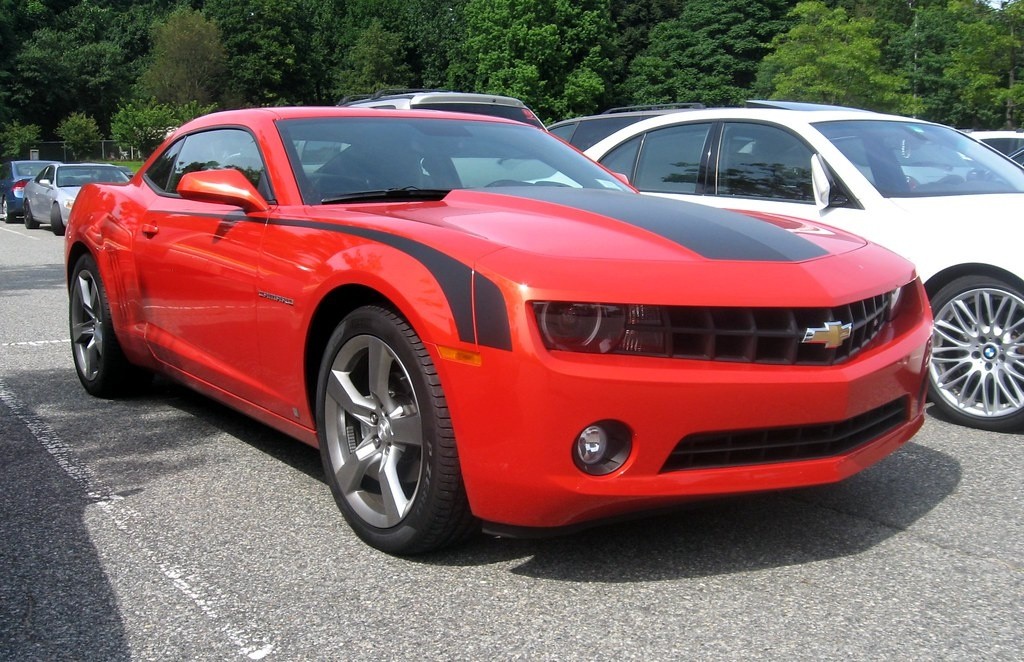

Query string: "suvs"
[[289, 87, 558, 196]]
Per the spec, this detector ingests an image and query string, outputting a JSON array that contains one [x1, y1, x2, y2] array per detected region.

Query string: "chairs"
[[304, 173, 372, 202], [727, 145, 815, 201], [19, 168, 34, 176], [63, 177, 77, 186]]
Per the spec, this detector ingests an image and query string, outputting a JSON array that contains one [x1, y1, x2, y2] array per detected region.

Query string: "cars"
[[537, 100, 1024, 205], [113, 165, 134, 179], [0, 160, 65, 225], [22, 162, 131, 236], [514, 108, 1024, 433], [63, 104, 934, 559]]
